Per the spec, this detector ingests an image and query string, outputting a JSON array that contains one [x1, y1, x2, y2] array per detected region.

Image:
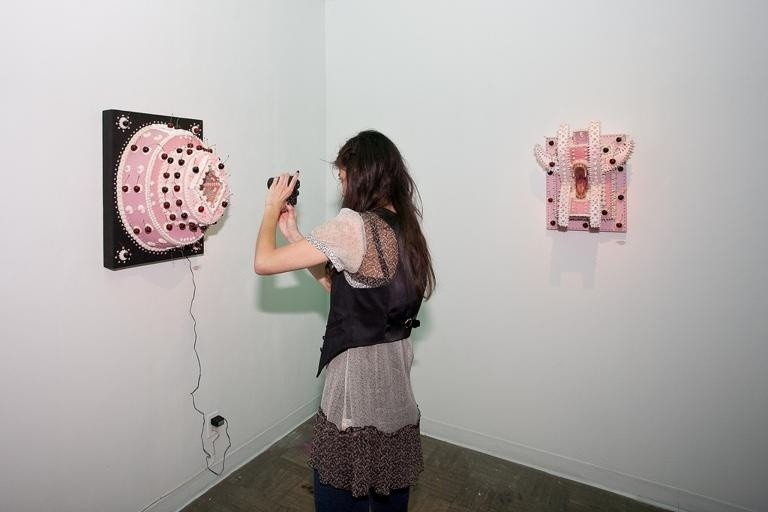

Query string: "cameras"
[[267, 175, 300, 206]]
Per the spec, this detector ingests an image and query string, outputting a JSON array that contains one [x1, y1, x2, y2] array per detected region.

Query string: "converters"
[[211, 415, 224, 427]]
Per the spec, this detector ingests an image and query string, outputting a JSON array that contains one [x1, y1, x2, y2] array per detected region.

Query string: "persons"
[[253, 130, 436, 511]]
